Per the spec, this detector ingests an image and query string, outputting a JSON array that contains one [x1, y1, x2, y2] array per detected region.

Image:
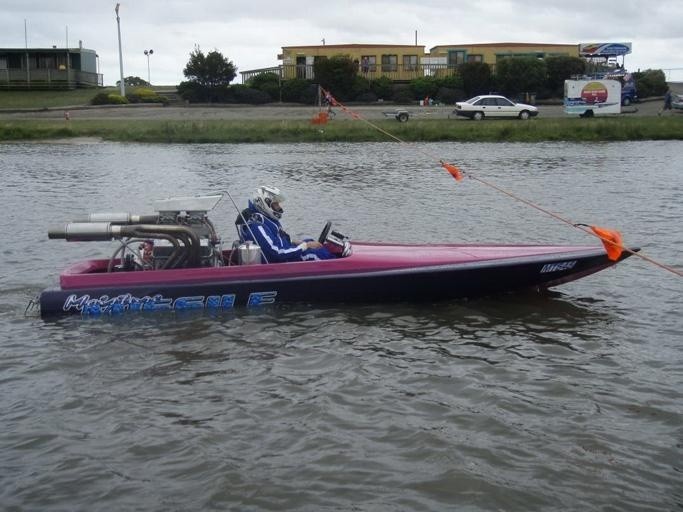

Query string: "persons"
[[656, 89, 675, 117], [240, 184, 337, 264]]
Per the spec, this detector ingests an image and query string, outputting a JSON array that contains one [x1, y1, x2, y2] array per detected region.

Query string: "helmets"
[[253, 184, 285, 221]]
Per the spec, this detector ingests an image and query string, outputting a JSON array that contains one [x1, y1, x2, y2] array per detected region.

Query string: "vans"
[[591, 72, 638, 106]]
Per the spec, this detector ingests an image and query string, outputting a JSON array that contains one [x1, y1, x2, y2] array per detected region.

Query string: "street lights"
[[115, 4, 125, 96], [143, 50, 153, 84]]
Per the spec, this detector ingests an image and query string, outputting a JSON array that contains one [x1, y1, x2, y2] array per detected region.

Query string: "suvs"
[[380, 108, 419, 122]]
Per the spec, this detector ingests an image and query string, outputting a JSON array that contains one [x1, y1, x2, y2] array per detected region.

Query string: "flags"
[[580, 226, 624, 262], [438, 165, 471, 181]]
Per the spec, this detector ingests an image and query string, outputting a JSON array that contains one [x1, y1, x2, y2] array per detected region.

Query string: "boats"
[[37, 208, 640, 318]]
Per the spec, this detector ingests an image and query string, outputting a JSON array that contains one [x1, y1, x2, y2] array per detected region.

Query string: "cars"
[[455, 95, 538, 121]]
